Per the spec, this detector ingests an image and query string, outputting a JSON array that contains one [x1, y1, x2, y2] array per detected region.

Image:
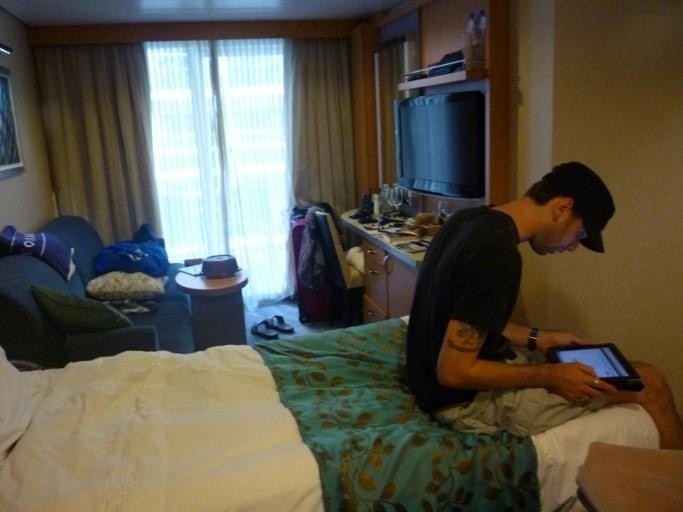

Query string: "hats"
[[543, 162, 614, 252]]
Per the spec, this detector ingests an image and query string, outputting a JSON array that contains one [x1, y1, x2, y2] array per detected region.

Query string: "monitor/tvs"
[[393, 89, 484, 198]]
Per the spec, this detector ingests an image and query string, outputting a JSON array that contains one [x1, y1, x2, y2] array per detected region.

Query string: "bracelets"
[[526, 326, 538, 353]]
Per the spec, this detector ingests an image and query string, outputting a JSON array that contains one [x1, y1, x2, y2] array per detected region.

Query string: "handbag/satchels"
[[298, 229, 326, 292]]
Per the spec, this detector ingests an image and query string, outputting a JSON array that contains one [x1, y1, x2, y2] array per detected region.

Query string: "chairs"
[[289, 206, 364, 328]]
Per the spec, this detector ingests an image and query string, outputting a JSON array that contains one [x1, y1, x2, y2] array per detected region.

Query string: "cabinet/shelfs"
[[363, 240, 419, 321]]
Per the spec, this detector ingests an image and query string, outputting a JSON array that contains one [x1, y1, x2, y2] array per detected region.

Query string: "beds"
[[0, 315, 663, 512]]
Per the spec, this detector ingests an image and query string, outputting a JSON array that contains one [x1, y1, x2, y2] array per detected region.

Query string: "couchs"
[[1, 215, 192, 371]]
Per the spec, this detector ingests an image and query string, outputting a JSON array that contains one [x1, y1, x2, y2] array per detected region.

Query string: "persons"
[[405, 159, 680, 452]]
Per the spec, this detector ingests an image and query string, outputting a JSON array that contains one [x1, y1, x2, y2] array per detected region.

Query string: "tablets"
[[547, 344, 638, 387]]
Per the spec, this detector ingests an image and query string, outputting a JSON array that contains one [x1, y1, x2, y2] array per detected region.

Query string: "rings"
[[592, 377, 599, 389], [581, 394, 589, 403]]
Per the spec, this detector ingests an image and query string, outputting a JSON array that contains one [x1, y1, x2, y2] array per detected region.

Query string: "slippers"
[[252, 316, 293, 339]]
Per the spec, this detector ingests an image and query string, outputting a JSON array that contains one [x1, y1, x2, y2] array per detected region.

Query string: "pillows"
[[32, 223, 169, 335]]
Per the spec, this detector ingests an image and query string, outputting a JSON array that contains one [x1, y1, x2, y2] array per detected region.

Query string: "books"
[[394, 238, 431, 254], [176, 261, 203, 276]]
